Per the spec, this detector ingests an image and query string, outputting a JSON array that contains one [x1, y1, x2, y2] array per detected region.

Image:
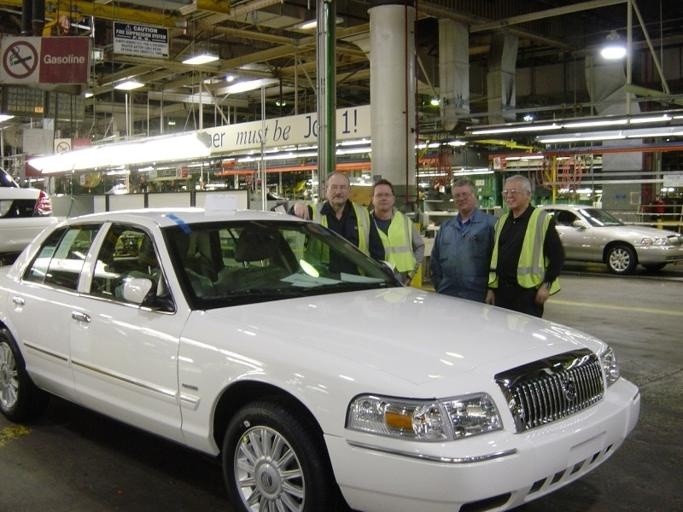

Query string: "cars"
[[0, 206, 642, 512], [0, 165, 58, 268], [539, 204, 683, 274]]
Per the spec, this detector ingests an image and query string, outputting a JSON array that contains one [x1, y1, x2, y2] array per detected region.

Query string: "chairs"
[[133, 226, 291, 293]]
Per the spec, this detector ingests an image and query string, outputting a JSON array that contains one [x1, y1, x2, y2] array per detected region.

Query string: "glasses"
[[501, 190, 525, 197]]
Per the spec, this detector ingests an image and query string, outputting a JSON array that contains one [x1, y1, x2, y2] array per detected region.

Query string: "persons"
[[486, 175, 566, 319], [287, 172, 385, 279], [428, 177, 498, 300], [369, 177, 425, 287]]
